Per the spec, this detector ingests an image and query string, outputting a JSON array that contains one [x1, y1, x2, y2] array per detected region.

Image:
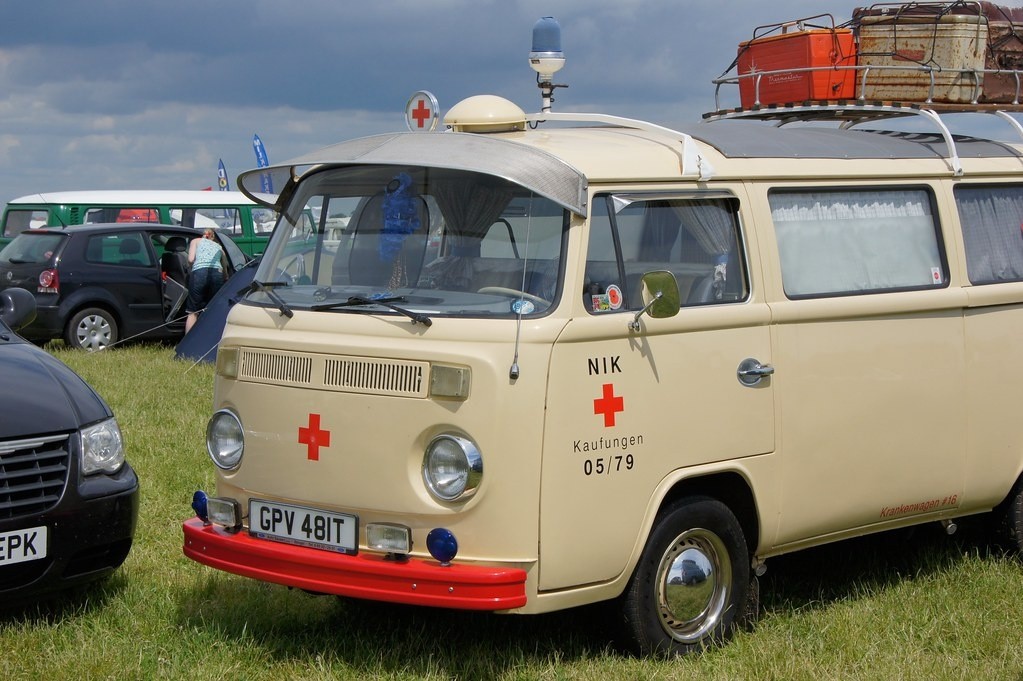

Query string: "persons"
[[186, 229, 228, 334]]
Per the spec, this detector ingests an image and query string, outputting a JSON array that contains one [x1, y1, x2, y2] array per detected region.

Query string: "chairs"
[[162, 237, 189, 287], [118, 239, 142, 265]]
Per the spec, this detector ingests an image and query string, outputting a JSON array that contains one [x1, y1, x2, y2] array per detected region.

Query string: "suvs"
[[0, 222, 294, 354]]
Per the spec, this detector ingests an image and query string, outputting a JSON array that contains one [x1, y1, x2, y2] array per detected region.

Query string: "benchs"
[[464, 258, 717, 309]]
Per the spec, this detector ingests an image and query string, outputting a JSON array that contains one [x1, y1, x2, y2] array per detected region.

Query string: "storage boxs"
[[737, 21, 857, 109], [856, 12, 990, 101]]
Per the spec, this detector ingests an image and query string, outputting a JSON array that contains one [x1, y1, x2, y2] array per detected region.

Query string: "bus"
[[0, 188, 326, 268]]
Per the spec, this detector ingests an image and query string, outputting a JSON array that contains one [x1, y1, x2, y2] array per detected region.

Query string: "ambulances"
[[178, 13, 1023, 666]]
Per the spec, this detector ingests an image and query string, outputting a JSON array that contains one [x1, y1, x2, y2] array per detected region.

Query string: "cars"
[[0, 287, 142, 605]]
